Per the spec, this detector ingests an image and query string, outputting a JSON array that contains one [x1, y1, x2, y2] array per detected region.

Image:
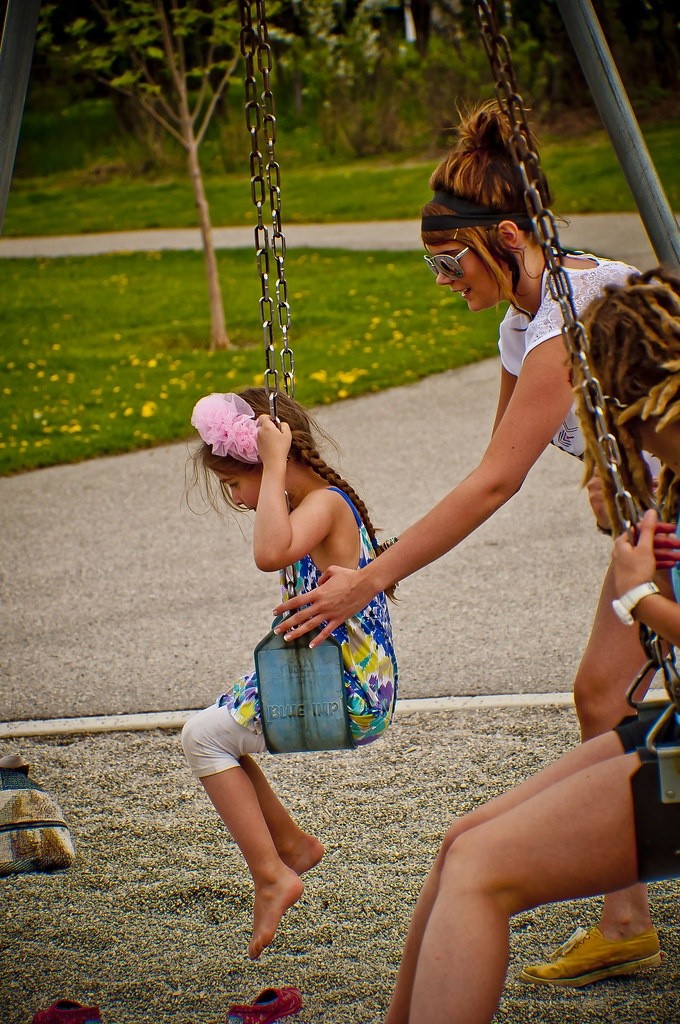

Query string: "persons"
[[383, 263, 680, 1023], [270, 97, 662, 985], [180, 385, 400, 959]]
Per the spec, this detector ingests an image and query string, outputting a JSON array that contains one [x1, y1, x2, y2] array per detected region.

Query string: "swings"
[[235, 1, 358, 757], [465, 0, 680, 888]]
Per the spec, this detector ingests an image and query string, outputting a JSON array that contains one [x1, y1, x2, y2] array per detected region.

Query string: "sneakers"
[[521, 927, 660, 986]]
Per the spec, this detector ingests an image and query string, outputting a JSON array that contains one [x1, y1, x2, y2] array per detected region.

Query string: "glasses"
[[424, 247, 471, 280]]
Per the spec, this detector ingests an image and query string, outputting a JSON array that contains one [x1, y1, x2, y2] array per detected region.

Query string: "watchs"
[[612, 581, 660, 627]]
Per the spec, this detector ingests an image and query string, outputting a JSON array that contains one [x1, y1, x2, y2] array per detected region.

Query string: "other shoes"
[[33, 999, 103, 1024], [228, 986, 302, 1024]]
